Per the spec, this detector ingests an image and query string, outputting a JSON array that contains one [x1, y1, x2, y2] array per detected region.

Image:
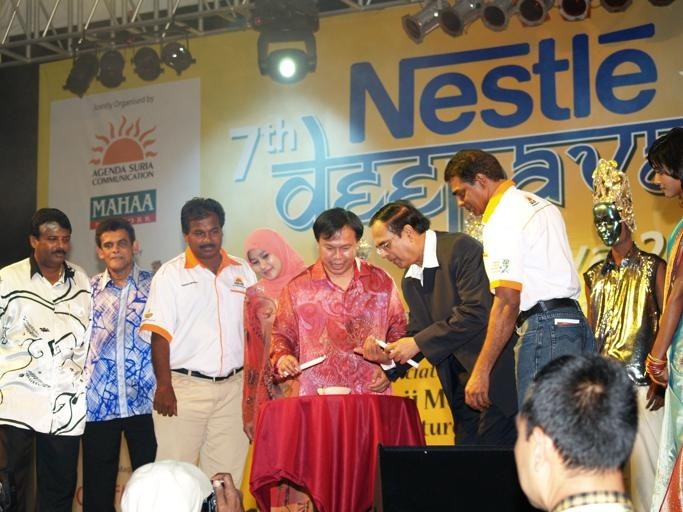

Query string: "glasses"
[[377, 241, 391, 254]]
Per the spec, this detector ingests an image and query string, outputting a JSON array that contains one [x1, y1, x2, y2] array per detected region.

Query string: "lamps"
[[481, 0, 513, 31], [159, 15, 195, 75], [517, 0, 555, 26], [400, 0, 451, 44], [62, 36, 99, 98], [96, 38, 125, 87], [558, 0, 589, 22], [130, 35, 163, 81], [439, 0, 489, 38], [247, 0, 320, 85], [599, 0, 631, 12]]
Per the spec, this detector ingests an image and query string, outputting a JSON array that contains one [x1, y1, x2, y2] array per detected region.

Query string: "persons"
[[137, 197, 258, 490], [444, 148, 600, 412], [583, 158, 667, 511], [243, 229, 313, 512], [119, 459, 244, 512], [643, 127, 683, 511], [269, 208, 409, 396], [353, 200, 518, 449], [81, 216, 158, 512], [0, 207, 94, 512], [514, 352, 638, 512]]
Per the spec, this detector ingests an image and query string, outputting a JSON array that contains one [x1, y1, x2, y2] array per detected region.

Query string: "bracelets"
[[644, 352, 670, 388]]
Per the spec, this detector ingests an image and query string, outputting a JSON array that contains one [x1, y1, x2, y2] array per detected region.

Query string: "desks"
[[250, 394, 426, 512]]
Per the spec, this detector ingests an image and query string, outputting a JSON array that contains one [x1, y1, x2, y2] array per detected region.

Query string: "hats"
[[592, 159, 637, 232], [120, 460, 214, 512]]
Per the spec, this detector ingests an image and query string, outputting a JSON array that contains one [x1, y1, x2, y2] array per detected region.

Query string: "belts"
[[170, 366, 244, 382], [514, 298, 580, 329]]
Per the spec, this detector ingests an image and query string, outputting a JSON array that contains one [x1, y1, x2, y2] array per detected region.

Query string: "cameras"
[[202, 479, 225, 512]]
